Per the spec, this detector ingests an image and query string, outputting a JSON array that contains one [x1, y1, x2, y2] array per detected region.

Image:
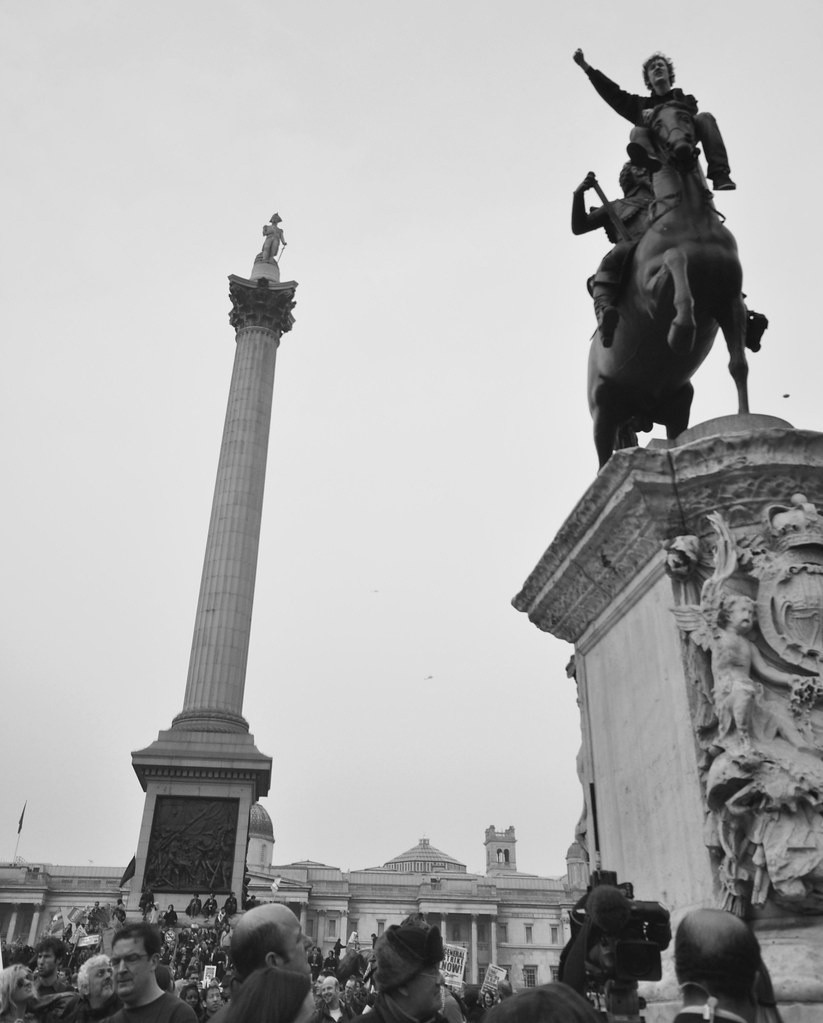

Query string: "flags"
[[17, 803, 26, 834], [119, 856, 135, 887], [271, 878, 281, 892]]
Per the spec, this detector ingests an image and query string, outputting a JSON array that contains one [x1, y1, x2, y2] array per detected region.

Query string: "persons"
[[87, 902, 101, 931], [164, 904, 177, 929], [245, 895, 256, 910], [224, 892, 238, 915], [189, 894, 202, 917], [138, 887, 154, 921], [201, 892, 217, 921], [111, 899, 126, 923], [574, 47, 735, 188], [0, 905, 608, 1022], [674, 908, 762, 1023], [262, 213, 287, 260], [573, 162, 767, 352], [702, 594, 823, 757]]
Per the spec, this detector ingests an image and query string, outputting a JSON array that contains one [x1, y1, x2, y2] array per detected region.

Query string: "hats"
[[482, 983, 606, 1023], [373, 913, 444, 990]]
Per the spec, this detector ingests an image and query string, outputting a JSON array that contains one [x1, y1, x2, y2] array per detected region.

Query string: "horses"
[[586, 96, 751, 474]]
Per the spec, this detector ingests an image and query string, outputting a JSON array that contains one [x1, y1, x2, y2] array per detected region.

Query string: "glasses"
[[109, 953, 148, 969], [17, 974, 32, 988]]
